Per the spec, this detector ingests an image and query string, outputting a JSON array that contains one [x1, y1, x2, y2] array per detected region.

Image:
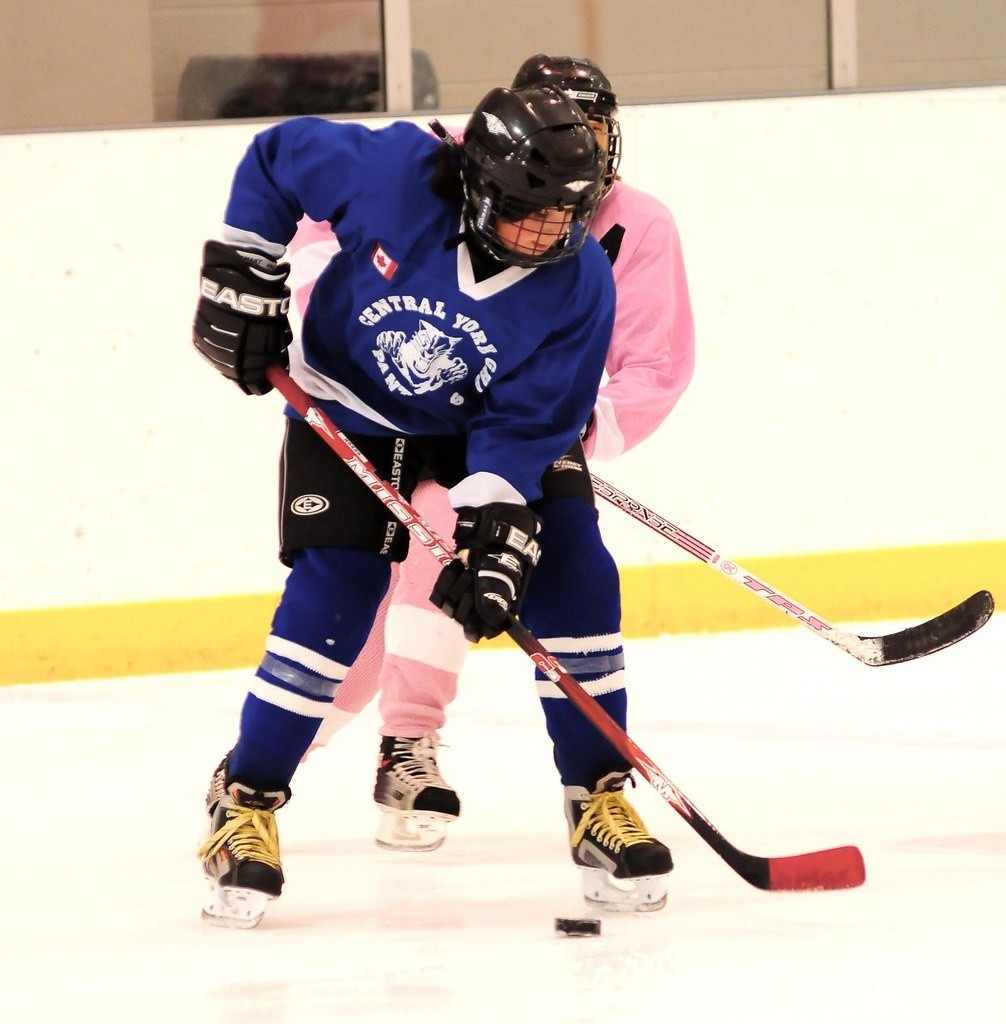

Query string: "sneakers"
[[565, 775, 670, 911], [198, 753, 286, 927], [370, 734, 461, 852]]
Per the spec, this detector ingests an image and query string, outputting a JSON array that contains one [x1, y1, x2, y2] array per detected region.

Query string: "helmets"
[[460, 83, 600, 269], [510, 54, 615, 187]]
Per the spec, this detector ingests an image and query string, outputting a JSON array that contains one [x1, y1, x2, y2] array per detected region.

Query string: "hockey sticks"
[[587, 472, 994, 666], [263, 360, 869, 890], [554, 917, 602, 937]]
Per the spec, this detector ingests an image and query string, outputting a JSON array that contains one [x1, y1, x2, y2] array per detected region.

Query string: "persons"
[[193, 84, 673, 929], [290, 53, 696, 854]]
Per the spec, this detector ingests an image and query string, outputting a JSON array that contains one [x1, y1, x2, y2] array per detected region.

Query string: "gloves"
[[192, 240, 293, 396], [431, 503, 542, 641]]
